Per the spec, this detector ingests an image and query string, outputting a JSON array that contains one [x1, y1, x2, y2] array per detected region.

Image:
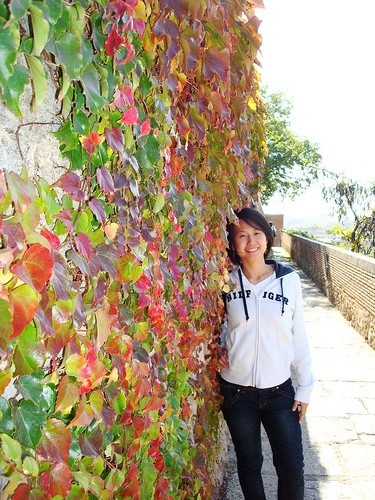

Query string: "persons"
[[268, 221, 276, 239], [217, 207, 315, 500]]
